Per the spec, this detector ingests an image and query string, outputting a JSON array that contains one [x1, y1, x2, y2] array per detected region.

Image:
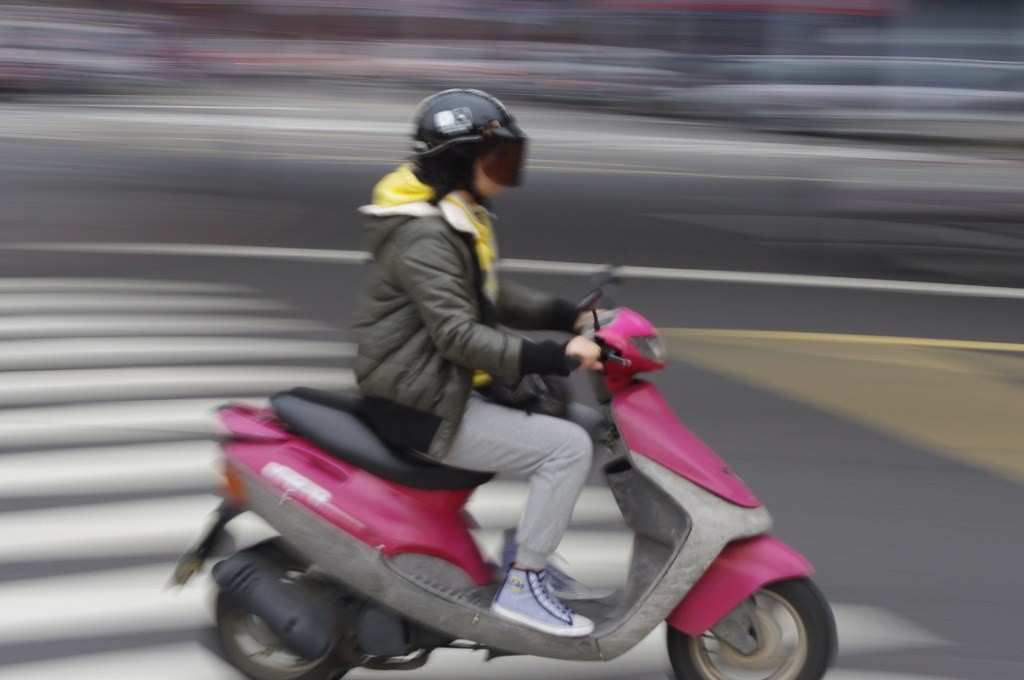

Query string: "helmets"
[[410, 88, 528, 187]]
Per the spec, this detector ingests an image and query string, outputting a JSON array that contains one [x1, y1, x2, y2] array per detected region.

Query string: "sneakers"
[[489, 560, 595, 637]]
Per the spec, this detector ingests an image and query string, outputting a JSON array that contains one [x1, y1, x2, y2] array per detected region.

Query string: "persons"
[[352, 88, 605, 636]]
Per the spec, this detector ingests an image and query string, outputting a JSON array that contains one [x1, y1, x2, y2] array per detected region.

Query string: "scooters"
[[165, 285, 837, 680]]
